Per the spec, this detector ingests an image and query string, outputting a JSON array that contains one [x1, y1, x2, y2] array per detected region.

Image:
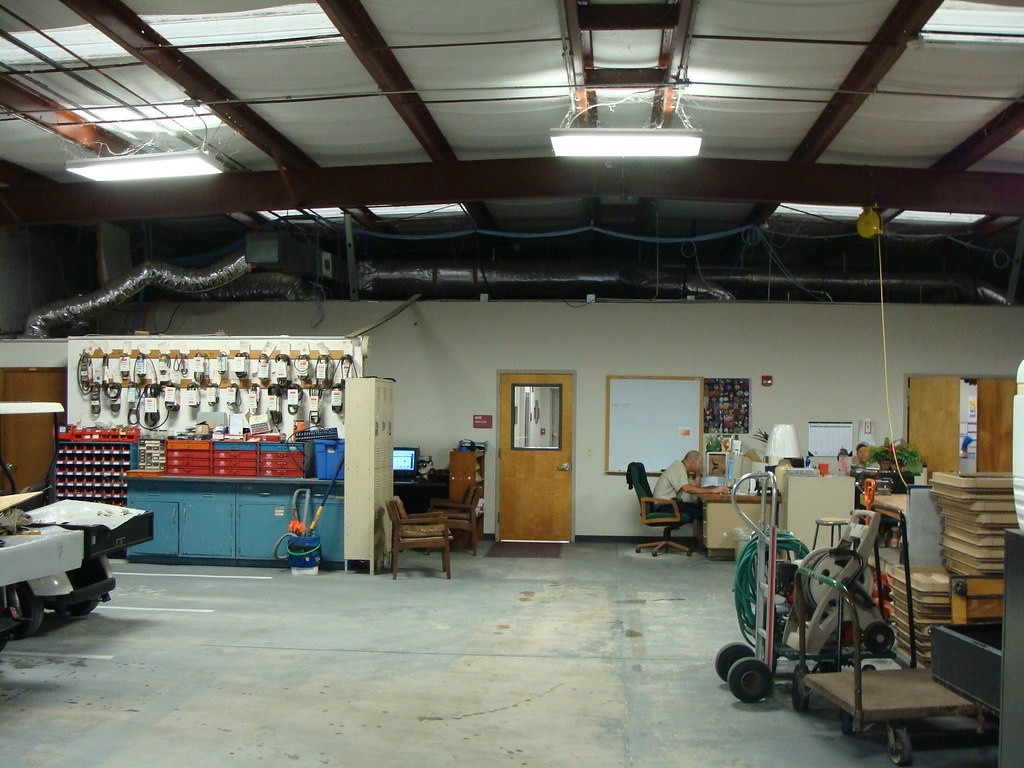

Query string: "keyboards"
[[394, 478, 421, 483]]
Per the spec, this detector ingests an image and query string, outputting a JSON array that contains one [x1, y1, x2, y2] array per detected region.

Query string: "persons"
[[653, 450, 728, 525], [418, 455, 434, 480], [856, 442, 881, 469]]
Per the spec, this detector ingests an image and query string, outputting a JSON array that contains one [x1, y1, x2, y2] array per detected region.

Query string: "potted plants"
[[874, 438, 919, 470]]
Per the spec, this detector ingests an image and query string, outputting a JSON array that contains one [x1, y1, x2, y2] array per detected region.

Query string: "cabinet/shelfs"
[[52, 378, 393, 577], [450, 450, 485, 541]]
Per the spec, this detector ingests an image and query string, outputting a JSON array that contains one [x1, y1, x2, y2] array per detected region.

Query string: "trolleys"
[[795, 568, 984, 766], [714, 470, 875, 703]]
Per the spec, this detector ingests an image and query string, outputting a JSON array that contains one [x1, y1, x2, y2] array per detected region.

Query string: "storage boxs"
[[930, 620, 1004, 718], [314, 438, 344, 480]]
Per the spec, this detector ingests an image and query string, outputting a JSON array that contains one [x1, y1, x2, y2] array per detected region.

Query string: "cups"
[[819, 464, 829, 475]]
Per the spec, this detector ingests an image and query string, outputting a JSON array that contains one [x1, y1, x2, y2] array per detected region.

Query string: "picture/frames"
[[707, 452, 729, 478]]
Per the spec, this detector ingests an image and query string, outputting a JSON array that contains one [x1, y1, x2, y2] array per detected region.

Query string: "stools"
[[813, 518, 850, 551]]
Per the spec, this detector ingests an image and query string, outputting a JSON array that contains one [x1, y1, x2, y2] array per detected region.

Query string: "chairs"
[[627, 462, 701, 557], [428, 484, 483, 557], [394, 481, 429, 515], [386, 494, 452, 581]]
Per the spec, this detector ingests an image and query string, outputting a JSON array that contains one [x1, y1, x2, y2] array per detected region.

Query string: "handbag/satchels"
[[287, 536, 323, 567]]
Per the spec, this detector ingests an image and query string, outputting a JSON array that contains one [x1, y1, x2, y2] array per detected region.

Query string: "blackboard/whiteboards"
[[605, 375, 704, 476]]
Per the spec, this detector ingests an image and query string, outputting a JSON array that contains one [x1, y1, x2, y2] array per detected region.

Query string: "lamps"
[[548, 81, 704, 158], [61, 98, 225, 184], [765, 424, 802, 498]]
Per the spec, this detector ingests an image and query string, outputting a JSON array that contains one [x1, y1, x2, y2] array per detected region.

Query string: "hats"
[[855, 442, 870, 450]]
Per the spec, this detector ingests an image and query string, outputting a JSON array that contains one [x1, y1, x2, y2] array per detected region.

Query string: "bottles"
[[840, 459, 846, 476], [885, 436, 889, 447], [865, 459, 869, 468], [721, 435, 732, 452], [837, 449, 847, 461], [806, 456, 810, 467], [734, 449, 738, 454], [732, 435, 741, 454]]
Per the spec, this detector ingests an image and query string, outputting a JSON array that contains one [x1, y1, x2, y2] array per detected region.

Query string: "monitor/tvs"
[[726, 454, 765, 495], [393, 447, 420, 476]]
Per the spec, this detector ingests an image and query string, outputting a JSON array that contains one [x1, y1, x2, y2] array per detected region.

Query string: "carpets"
[[486, 541, 561, 557]]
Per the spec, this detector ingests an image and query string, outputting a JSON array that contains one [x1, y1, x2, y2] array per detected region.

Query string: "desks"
[[695, 489, 782, 561], [782, 475, 855, 564], [860, 493, 909, 566]]
